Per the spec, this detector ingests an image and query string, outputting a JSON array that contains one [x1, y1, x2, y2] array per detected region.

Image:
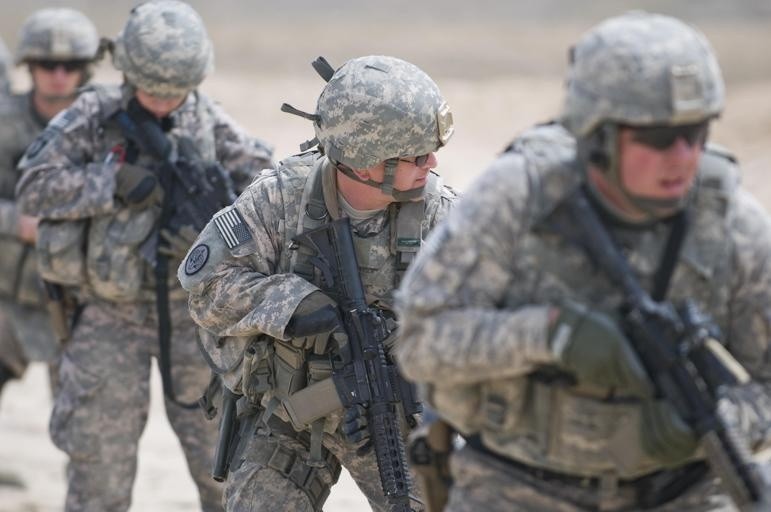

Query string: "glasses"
[[36, 62, 86, 71], [614, 121, 710, 153], [396, 153, 432, 169]]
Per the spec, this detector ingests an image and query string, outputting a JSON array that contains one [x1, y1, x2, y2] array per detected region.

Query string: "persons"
[[0, 5, 110, 395], [168, 49, 469, 511], [6, 0, 282, 512], [386, 5, 771, 512]]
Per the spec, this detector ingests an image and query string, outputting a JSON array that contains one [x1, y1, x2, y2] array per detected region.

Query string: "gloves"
[[639, 395, 701, 465], [547, 300, 652, 403], [287, 286, 350, 356], [115, 161, 164, 213]]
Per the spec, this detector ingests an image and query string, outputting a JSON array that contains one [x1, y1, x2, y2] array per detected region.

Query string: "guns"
[[213, 386, 242, 483], [283, 215, 422, 512], [563, 186, 770, 512], [101, 96, 239, 234]]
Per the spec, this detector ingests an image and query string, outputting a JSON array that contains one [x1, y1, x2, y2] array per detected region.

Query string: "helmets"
[[14, 10, 100, 66], [111, 0, 215, 96], [313, 56, 455, 168], [562, 12, 726, 140]]
